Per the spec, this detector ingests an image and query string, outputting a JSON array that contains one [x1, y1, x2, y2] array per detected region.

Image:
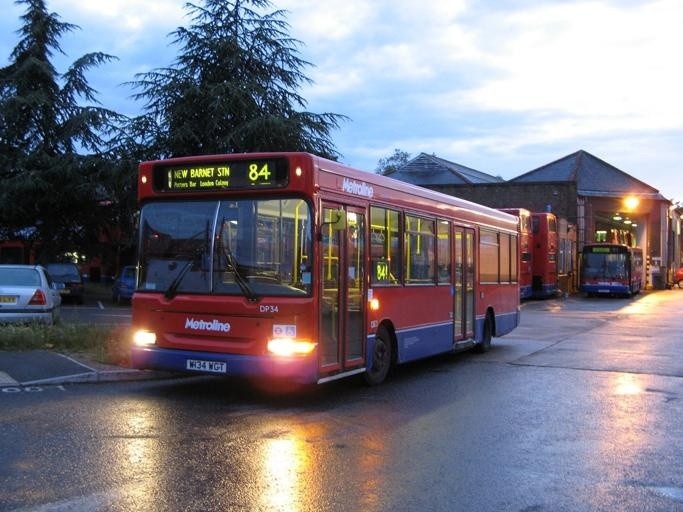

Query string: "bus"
[[583, 244, 644, 295], [529, 212, 562, 300], [132, 152, 522, 386], [494, 208, 535, 298]]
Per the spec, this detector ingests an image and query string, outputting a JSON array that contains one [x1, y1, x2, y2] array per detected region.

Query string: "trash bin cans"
[[89, 267, 100, 279], [652, 271, 665, 289]]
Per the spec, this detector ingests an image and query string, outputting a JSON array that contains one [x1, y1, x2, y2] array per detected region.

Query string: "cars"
[[0, 261, 137, 325]]
